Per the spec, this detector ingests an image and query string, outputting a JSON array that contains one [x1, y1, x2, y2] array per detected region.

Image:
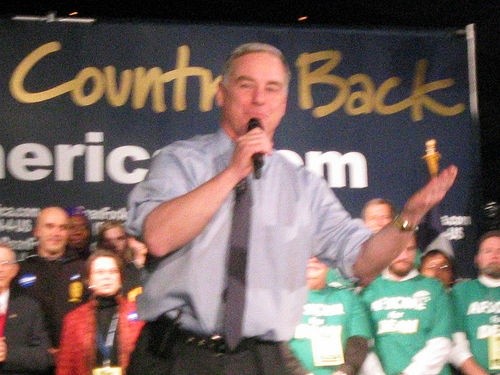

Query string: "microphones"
[[247, 118, 265, 169]]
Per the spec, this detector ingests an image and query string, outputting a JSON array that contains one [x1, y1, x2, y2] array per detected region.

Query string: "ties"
[[0, 310, 7, 339], [220, 177, 254, 352]]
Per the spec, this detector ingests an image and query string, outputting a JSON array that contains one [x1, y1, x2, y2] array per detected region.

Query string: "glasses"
[[424, 263, 451, 273], [0, 259, 19, 267]]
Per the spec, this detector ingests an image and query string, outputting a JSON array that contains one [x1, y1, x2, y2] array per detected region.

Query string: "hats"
[[65, 204, 91, 226]]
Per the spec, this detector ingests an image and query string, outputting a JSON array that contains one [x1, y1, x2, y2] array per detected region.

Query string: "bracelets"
[[392, 213, 418, 234]]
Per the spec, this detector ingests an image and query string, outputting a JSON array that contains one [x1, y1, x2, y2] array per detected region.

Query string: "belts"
[[178, 329, 271, 354]]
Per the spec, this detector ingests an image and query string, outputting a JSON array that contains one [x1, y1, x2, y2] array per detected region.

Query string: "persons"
[[124, 42, 458, 375], [0, 197, 500, 375]]
